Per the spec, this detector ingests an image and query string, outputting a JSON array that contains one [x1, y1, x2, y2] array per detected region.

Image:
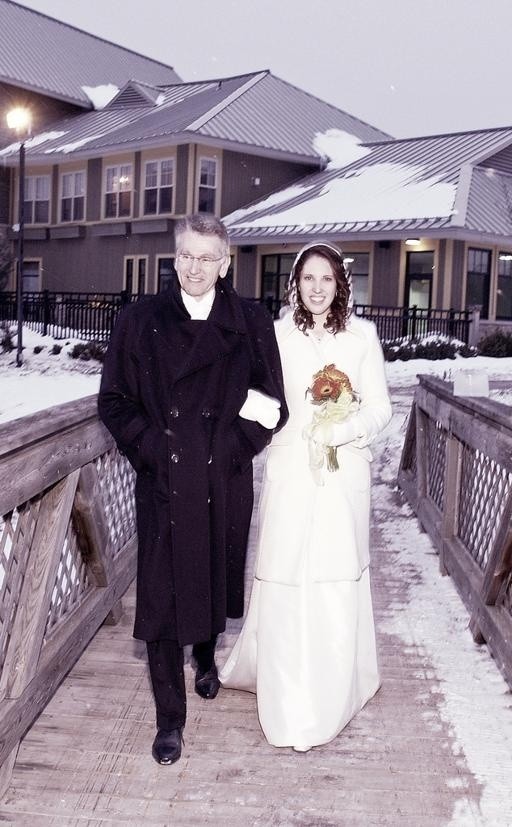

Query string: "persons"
[[96, 209, 292, 768], [214, 238, 395, 752]]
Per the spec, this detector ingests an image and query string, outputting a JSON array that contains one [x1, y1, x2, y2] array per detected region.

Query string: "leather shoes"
[[193, 665, 221, 701], [151, 728, 186, 766]]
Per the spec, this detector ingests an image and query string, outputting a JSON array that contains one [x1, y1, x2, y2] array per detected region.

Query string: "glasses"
[[176, 252, 228, 266]]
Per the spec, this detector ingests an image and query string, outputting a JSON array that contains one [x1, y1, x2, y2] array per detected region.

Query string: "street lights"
[[6, 104, 36, 371]]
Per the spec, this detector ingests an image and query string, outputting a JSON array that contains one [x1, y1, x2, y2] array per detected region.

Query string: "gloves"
[[317, 421, 359, 449], [237, 389, 282, 430]]
